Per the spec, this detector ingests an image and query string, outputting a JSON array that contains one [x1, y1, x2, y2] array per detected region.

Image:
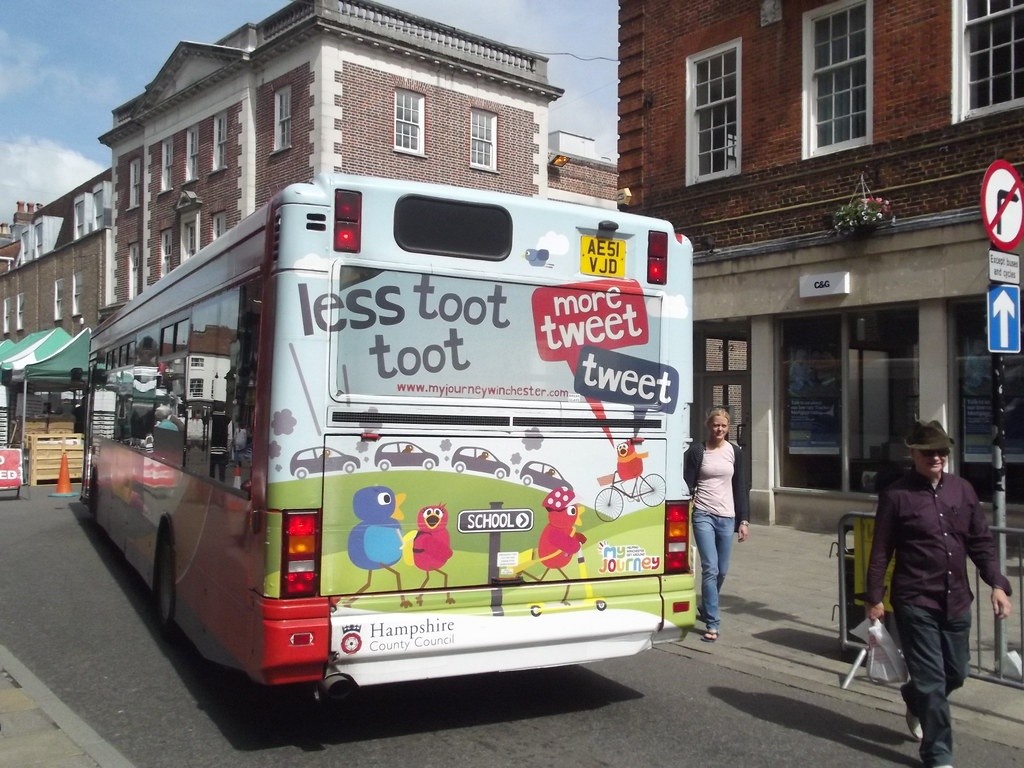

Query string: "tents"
[[0, 338, 15, 357], [12, 325, 134, 451], [0, 325, 74, 386]]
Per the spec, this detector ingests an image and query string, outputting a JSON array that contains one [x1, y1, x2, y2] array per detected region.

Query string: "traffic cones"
[[234, 467, 242, 489], [48, 450, 80, 499]]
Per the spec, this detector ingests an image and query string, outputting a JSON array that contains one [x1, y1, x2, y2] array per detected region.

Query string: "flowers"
[[833, 198, 897, 237]]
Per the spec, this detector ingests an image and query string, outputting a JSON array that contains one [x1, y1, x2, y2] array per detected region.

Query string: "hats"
[[904, 420, 955, 449]]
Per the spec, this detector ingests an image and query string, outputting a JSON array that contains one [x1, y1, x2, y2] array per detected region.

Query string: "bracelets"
[[739, 522, 749, 527]]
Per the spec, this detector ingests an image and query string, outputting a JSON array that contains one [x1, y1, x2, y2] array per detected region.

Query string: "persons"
[[867, 418, 1013, 768], [225, 310, 261, 467], [202, 401, 232, 483], [684, 405, 749, 642], [155, 406, 184, 432], [75, 397, 86, 433]]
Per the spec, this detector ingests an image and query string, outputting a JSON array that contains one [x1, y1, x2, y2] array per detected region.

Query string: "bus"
[[80, 173, 695, 707]]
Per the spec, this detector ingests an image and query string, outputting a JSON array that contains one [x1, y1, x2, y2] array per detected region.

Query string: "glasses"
[[918, 448, 950, 457]]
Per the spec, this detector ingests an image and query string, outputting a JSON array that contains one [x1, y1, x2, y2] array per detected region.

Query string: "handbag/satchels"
[[865, 619, 908, 684]]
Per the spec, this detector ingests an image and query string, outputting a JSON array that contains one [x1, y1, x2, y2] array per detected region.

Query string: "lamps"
[[547, 154, 572, 168]]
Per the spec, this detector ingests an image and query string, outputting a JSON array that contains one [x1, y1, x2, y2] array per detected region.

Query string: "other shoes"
[[932, 765, 953, 768], [905, 706, 923, 739]]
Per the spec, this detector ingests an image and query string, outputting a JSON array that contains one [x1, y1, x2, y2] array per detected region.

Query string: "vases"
[[849, 225, 877, 236]]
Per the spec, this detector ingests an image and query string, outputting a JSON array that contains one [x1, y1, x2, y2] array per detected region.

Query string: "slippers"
[[697, 603, 704, 617], [701, 631, 720, 642]]
